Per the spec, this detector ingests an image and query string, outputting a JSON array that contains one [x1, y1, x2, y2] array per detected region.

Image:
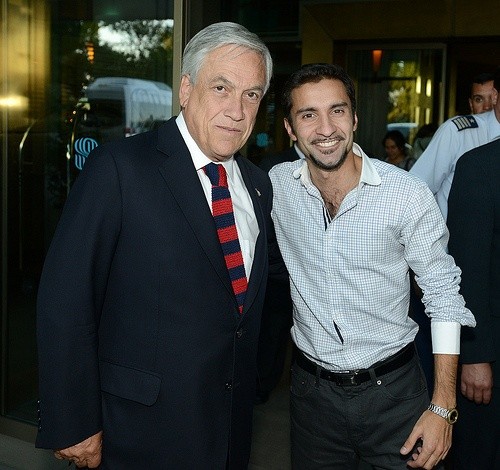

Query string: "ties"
[[203, 162, 247, 313]]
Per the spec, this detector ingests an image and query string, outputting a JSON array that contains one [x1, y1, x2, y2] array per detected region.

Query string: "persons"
[[406, 59, 500, 400], [381, 128, 418, 172], [467, 69, 496, 115], [445, 138, 500, 470], [34, 21, 289, 470], [267, 62, 478, 470]]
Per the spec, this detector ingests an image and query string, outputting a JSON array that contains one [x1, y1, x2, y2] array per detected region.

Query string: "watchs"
[[427, 402, 460, 426]]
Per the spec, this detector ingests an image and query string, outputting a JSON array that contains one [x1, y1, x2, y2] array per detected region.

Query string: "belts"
[[297, 344, 415, 386]]
